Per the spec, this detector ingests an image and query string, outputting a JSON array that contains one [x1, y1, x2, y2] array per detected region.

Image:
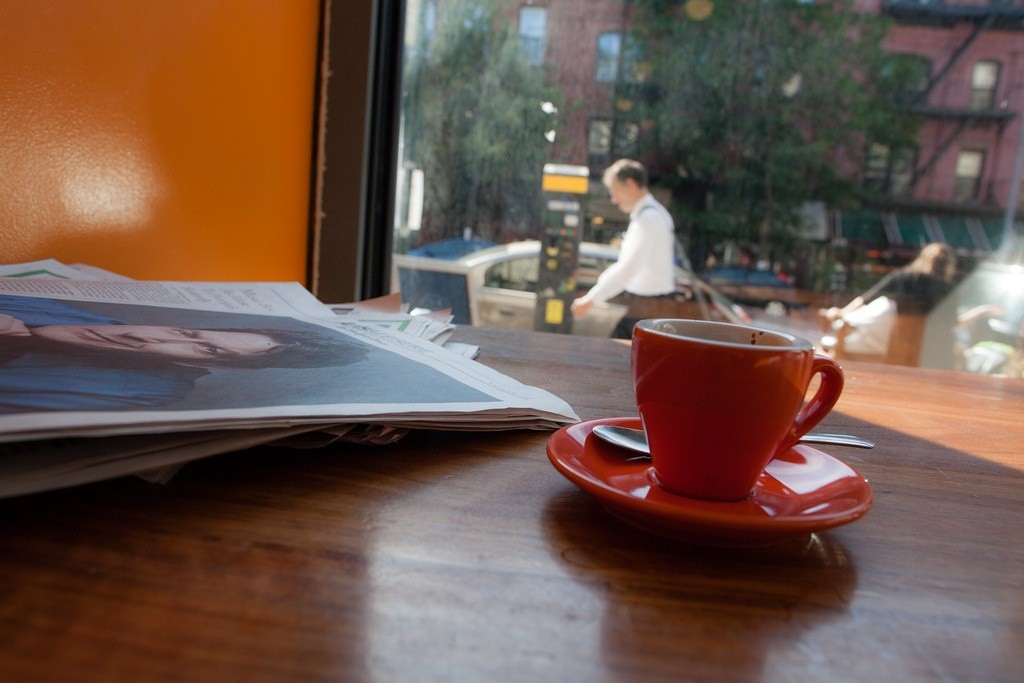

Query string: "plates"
[[546, 416, 874, 547]]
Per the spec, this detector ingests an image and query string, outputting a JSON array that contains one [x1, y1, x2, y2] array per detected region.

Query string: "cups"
[[630, 318, 845, 502]]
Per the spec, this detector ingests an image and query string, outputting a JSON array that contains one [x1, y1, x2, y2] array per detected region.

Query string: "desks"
[[0, 319, 1024, 683]]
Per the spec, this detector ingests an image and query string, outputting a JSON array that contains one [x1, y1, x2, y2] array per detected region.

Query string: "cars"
[[448, 241, 746, 336]]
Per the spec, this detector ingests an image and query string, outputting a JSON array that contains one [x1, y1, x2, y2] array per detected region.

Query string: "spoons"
[[592, 425, 875, 454]]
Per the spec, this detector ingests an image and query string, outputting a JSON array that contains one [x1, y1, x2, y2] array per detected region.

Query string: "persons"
[[831, 243, 957, 368], [1, 296, 370, 411], [570, 159, 675, 340]]
